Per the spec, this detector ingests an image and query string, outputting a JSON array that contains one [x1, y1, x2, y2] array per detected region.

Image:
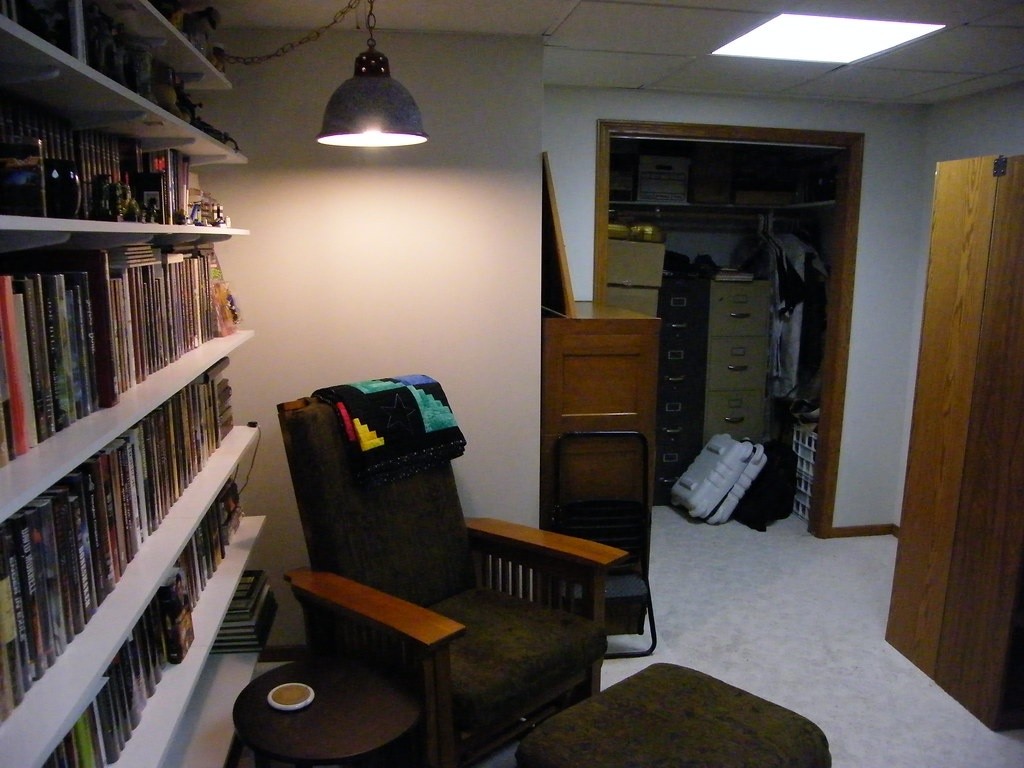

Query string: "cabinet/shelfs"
[[653, 274, 709, 504], [0, 0, 275, 768], [540, 301, 662, 516], [704, 279, 771, 443]]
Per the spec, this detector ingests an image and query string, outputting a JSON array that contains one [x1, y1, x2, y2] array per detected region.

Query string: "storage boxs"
[[636, 154, 690, 203], [791, 426, 818, 478], [793, 470, 813, 524], [610, 153, 634, 201], [605, 288, 658, 318], [608, 238, 665, 288]]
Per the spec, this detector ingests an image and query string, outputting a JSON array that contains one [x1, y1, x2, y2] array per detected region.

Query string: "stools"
[[514, 663, 831, 768]]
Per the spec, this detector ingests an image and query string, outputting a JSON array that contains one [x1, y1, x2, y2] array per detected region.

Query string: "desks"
[[233, 659, 424, 768]]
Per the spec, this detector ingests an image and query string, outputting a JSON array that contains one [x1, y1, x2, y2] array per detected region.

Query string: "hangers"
[[754, 213, 800, 256]]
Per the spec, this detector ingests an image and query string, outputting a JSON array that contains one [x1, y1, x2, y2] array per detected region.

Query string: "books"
[[0, 243, 241, 768], [210, 569, 279, 654], [0, 0, 191, 224]]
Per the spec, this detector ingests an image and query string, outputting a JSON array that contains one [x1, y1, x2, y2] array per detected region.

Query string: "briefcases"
[[671, 433, 767, 524]]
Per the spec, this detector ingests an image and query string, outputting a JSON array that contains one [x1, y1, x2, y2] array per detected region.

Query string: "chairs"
[[547, 429, 658, 660], [276, 374, 630, 768]]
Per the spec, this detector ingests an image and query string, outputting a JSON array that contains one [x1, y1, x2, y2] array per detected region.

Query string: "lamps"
[[313, 0, 430, 148]]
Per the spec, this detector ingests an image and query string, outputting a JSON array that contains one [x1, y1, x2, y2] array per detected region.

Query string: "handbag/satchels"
[[730, 439, 799, 532]]
